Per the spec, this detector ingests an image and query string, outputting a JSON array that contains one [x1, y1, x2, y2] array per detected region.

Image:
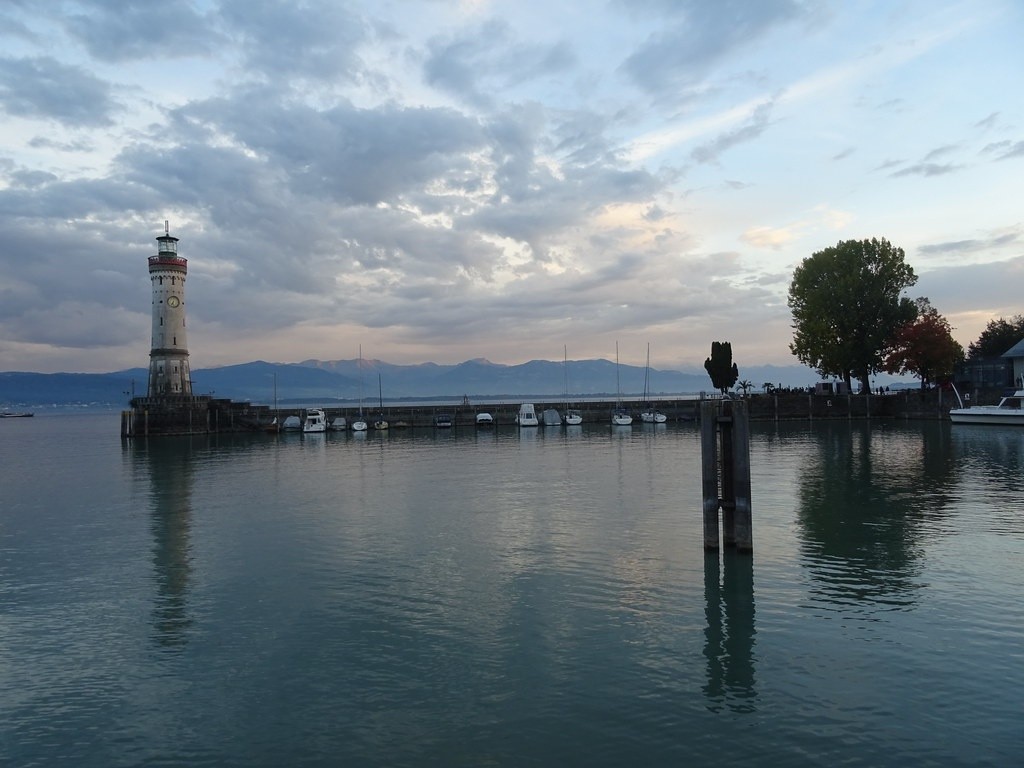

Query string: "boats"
[[949, 406, 1024, 424], [641, 412, 667, 422], [436, 415, 452, 427], [303, 410, 330, 431], [612, 415, 633, 425], [565, 410, 582, 424], [515, 404, 539, 426], [477, 413, 492, 423], [0, 412, 35, 417], [375, 421, 388, 430]]
